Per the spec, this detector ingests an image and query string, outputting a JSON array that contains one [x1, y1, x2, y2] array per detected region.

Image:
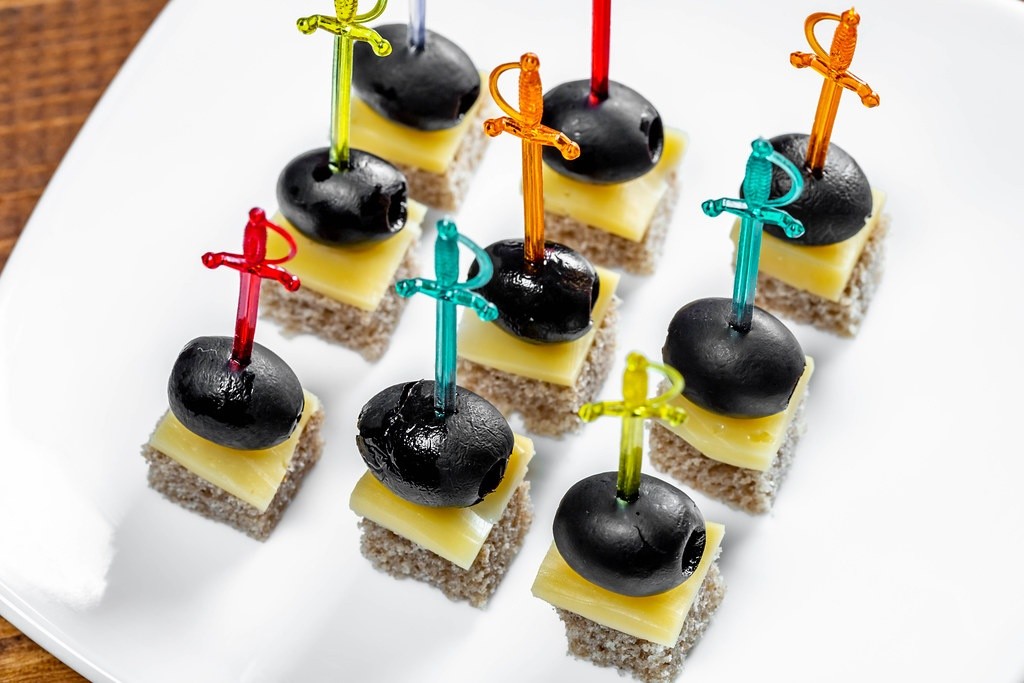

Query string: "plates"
[[0, 0, 1024, 683]]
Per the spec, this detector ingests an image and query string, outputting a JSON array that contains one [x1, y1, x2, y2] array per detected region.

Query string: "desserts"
[[137, 0, 888, 683]]
